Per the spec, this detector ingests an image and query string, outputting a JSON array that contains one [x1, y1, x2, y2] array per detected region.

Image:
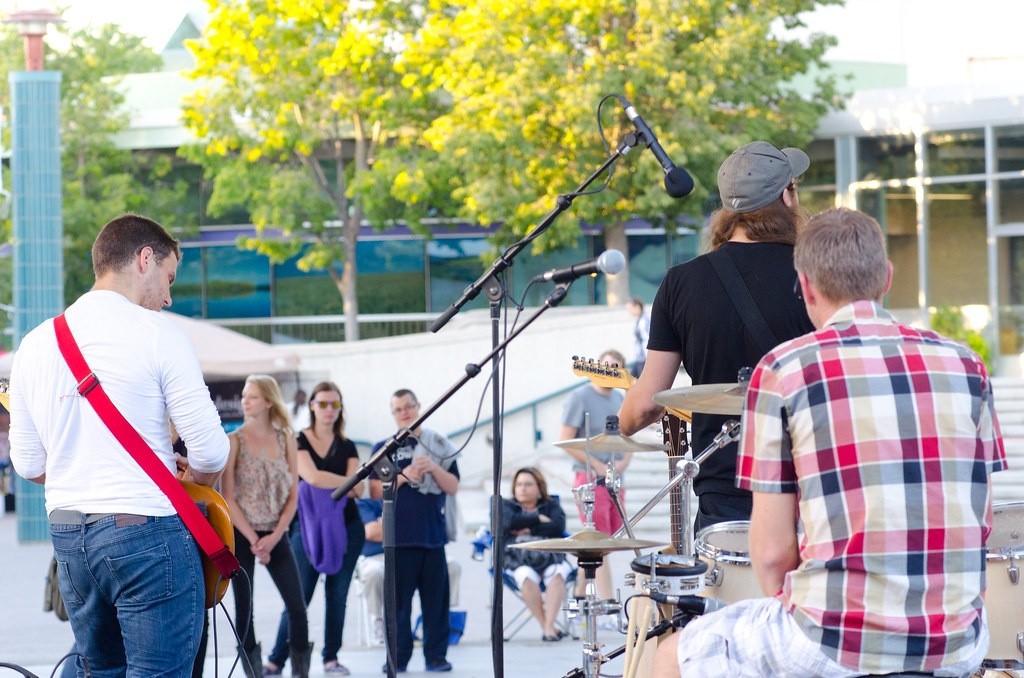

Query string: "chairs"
[[471, 496, 581, 642]]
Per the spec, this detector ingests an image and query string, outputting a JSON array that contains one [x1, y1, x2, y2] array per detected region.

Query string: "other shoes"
[[383, 665, 408, 672], [324, 665, 349, 676], [426, 661, 452, 672], [262, 667, 282, 678], [557, 631, 567, 639], [542, 636, 559, 645]]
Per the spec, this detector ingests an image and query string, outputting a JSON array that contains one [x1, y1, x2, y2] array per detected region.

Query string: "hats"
[[717, 139, 811, 214]]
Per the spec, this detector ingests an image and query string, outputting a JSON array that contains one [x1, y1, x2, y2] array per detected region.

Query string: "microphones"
[[614, 91, 695, 199], [649, 591, 726, 616], [533, 249, 626, 283]]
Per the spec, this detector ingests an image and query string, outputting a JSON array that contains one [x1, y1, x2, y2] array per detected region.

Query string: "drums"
[[979, 499, 1024, 668], [695, 518, 777, 609]]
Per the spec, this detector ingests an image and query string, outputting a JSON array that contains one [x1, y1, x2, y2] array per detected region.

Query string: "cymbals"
[[650, 382, 748, 417], [553, 433, 668, 452], [506, 527, 671, 558]]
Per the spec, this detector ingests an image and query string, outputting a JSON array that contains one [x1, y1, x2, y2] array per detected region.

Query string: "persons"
[[495, 468, 568, 643], [647, 207, 1010, 678], [0, 376, 15, 515], [559, 348, 633, 610], [260, 381, 365, 676], [616, 140, 819, 556], [353, 389, 461, 674], [221, 374, 308, 678], [9, 214, 230, 678], [624, 298, 649, 378]]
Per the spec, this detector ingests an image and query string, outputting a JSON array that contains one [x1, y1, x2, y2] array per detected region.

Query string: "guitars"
[[571, 352, 692, 421], [0, 369, 235, 609]]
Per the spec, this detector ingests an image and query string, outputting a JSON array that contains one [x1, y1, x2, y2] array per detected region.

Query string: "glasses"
[[312, 400, 341, 410]]
